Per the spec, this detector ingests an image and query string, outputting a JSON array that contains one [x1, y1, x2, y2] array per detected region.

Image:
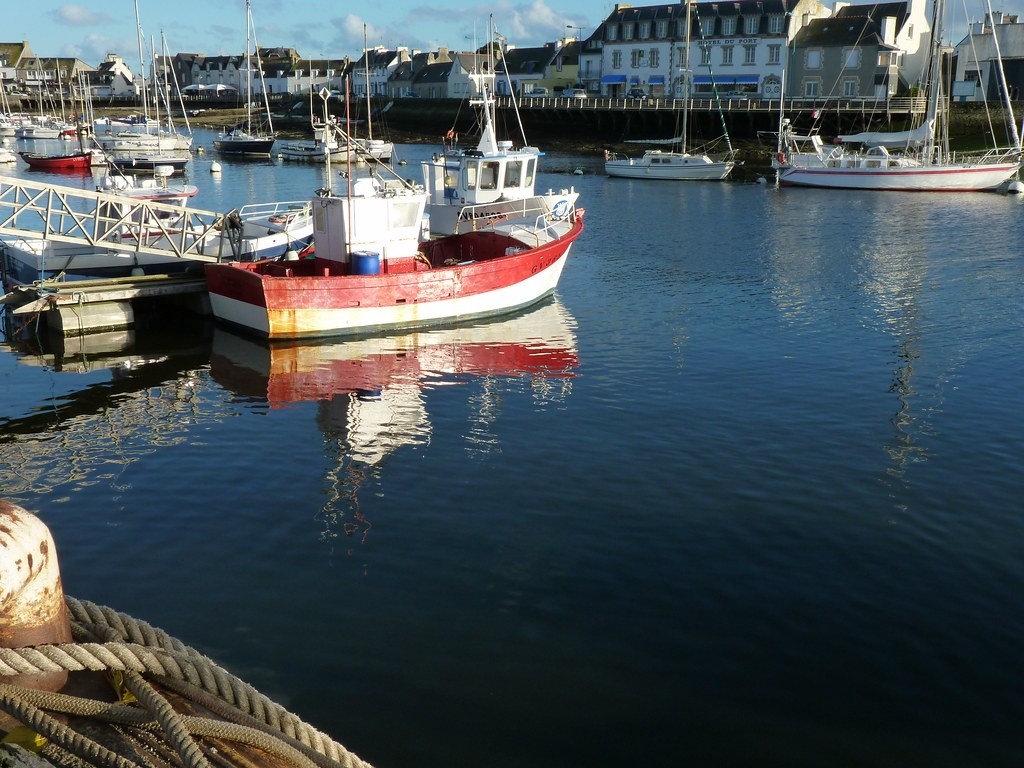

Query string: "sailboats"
[[604, 0, 736, 180], [770, 0, 1024, 191], [212, 0, 278, 158], [342, 22, 394, 164], [0, 0, 196, 175]]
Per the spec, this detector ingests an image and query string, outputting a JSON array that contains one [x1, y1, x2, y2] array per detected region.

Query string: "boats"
[[420, 10, 579, 235], [0, 135, 315, 291], [208, 296, 582, 543], [278, 57, 373, 164], [204, 68, 585, 339]]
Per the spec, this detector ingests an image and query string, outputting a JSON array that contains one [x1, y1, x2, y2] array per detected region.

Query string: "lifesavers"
[[776, 153, 785, 164], [446, 130, 455, 139], [269, 214, 293, 223]]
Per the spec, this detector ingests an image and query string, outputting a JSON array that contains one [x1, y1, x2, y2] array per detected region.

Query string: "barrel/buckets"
[[352, 252, 379, 276]]
